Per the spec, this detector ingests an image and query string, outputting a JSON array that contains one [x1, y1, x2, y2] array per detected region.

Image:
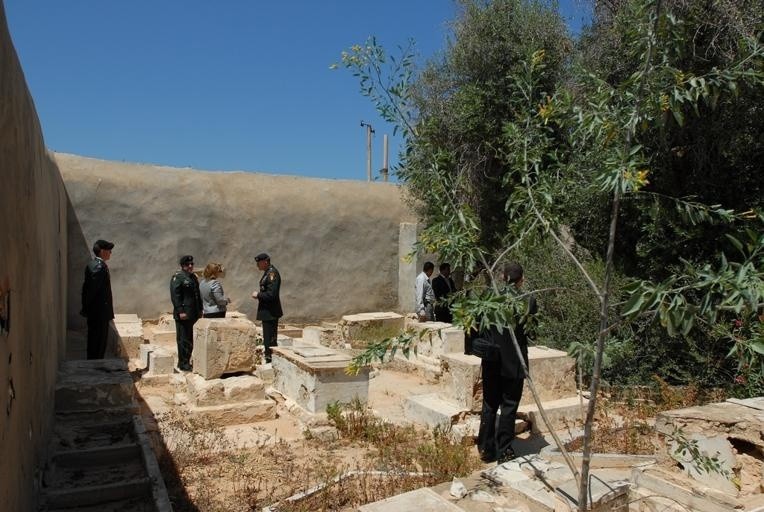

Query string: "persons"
[[199, 263, 231, 318], [250, 254, 284, 364], [414, 262, 438, 321], [431, 262, 458, 324], [77, 240, 114, 360], [476, 261, 537, 465], [169, 255, 203, 373]]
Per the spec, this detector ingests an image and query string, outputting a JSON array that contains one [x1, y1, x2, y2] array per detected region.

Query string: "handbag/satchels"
[[464, 317, 494, 360]]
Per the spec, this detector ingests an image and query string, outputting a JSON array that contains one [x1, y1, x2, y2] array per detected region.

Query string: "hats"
[[93, 240, 114, 253], [255, 253, 270, 262], [504, 263, 522, 280], [180, 255, 193, 264]]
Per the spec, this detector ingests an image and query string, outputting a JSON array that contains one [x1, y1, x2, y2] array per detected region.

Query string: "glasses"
[[184, 262, 194, 266]]
